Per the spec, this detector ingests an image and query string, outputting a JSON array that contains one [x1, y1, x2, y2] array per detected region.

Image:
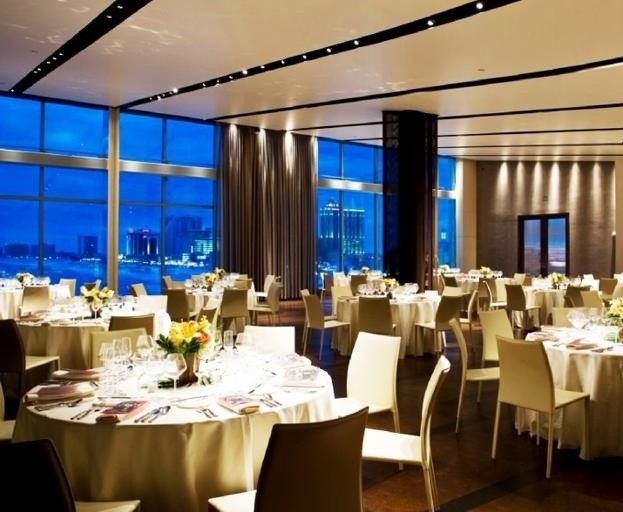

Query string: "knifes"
[[245, 381, 262, 394]]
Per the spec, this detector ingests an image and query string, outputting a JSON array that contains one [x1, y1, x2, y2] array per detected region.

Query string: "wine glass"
[[52, 294, 146, 325], [235, 332, 250, 364], [568, 306, 621, 346], [358, 283, 418, 302], [165, 353, 187, 400], [213, 330, 233, 361], [456, 270, 502, 280], [192, 352, 207, 394], [98, 335, 131, 397], [147, 354, 164, 399], [136, 335, 154, 370]]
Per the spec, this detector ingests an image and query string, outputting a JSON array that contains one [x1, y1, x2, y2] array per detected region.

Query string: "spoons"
[[134, 404, 169, 423], [34, 398, 82, 411]]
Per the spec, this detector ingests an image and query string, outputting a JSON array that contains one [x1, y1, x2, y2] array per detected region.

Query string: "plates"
[[261, 355, 310, 367], [28, 389, 92, 399], [50, 369, 105, 378]]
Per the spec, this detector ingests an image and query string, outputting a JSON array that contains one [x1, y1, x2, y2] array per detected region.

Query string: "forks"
[[196, 406, 218, 418], [259, 393, 283, 408], [71, 402, 103, 419]]
